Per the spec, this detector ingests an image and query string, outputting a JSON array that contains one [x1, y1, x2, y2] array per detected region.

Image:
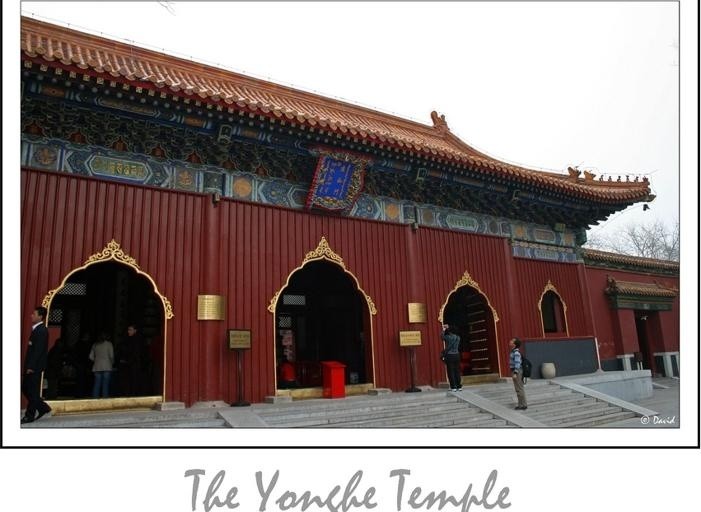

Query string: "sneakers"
[[456, 386, 462, 391], [449, 387, 457, 392]]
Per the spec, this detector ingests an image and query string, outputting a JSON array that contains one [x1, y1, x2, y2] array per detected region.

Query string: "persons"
[[21, 307, 52, 424], [509, 337, 528, 410], [45, 322, 153, 398], [277, 350, 298, 389], [441, 323, 465, 392]]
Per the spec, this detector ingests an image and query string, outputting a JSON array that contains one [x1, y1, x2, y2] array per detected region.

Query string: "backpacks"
[[518, 351, 531, 377]]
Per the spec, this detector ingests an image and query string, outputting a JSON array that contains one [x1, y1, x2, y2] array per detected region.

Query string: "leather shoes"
[[516, 406, 527, 410]]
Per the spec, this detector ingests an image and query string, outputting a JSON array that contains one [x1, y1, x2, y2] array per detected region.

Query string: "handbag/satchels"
[[440, 351, 448, 364]]
